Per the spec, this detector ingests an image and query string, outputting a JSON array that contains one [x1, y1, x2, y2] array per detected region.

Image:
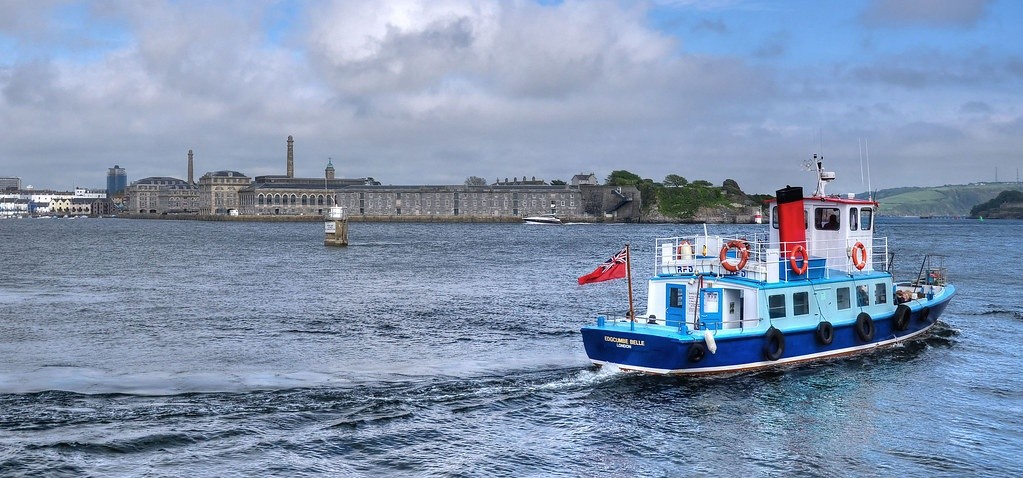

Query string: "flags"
[[578, 247, 627, 284]]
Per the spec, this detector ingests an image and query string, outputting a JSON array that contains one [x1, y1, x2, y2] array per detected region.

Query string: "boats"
[[522, 212, 563, 225], [579, 136, 958, 382], [976, 215, 985, 225], [323, 202, 349, 248]]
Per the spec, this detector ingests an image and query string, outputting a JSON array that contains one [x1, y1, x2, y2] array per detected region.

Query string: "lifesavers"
[[686, 344, 706, 364], [677, 241, 693, 260], [855, 311, 875, 343], [893, 304, 912, 332], [816, 321, 834, 345], [852, 241, 867, 270], [920, 306, 930, 322], [719, 240, 748, 273], [764, 328, 785, 362], [790, 245, 809, 275]]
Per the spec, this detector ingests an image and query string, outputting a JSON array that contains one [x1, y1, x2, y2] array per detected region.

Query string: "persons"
[[823, 214, 839, 230]]
